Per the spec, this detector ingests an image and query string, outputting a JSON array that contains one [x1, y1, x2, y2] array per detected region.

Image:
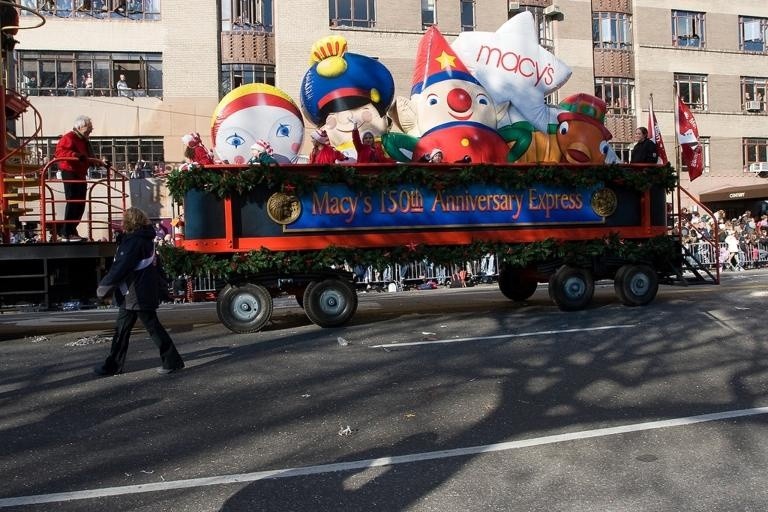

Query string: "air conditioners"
[[746, 101, 760, 111], [750, 161, 768, 174]]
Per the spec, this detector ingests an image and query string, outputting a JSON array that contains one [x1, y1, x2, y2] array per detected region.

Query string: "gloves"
[[77, 153, 87, 160], [104, 161, 110, 167]]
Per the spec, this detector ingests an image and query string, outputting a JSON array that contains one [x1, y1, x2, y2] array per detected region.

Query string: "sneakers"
[[158, 367, 175, 374], [61, 235, 86, 242], [96, 366, 123, 375]]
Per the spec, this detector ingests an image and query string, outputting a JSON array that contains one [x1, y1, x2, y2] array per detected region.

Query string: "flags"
[[648, 102, 668, 165], [675, 94, 702, 182]]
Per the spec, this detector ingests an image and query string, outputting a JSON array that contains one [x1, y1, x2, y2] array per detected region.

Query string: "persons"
[[178, 131, 215, 171], [92, 205, 185, 376], [239, 9, 249, 23], [383, 24, 533, 164], [7, 71, 173, 245], [331, 251, 498, 293], [497, 92, 622, 164], [247, 140, 278, 171], [352, 119, 402, 164], [625, 128, 657, 165], [417, 147, 470, 164], [211, 82, 308, 163], [299, 36, 404, 164], [308, 127, 354, 166], [667, 202, 767, 281], [11, 0, 155, 19]]
